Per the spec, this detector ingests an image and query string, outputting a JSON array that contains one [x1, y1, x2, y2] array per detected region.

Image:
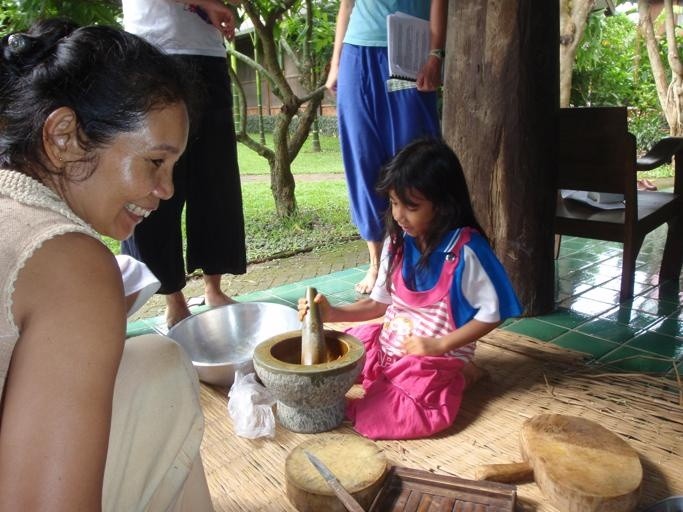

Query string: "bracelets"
[[430, 48, 445, 60]]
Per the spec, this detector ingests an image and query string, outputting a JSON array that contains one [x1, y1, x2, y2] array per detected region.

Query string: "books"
[[386, 10, 444, 85]]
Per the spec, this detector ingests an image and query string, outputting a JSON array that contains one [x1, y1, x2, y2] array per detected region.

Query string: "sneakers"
[[637, 179, 657, 191]]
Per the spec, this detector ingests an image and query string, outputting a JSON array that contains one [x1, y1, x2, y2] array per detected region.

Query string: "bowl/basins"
[[165, 302, 303, 387]]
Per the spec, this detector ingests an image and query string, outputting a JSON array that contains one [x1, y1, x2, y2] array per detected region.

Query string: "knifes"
[[305, 449, 366, 512]]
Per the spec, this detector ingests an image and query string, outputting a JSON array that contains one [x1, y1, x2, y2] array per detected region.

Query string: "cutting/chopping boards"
[[519, 412, 643, 511], [285, 431, 389, 512]]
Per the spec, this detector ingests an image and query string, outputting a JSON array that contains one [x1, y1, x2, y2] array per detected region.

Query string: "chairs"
[[553, 106, 682, 297]]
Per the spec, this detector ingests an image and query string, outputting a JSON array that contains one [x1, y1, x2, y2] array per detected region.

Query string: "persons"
[[296, 135, 523, 441], [0, 16, 221, 511], [325, 0, 450, 296], [119, 0, 248, 329]]
